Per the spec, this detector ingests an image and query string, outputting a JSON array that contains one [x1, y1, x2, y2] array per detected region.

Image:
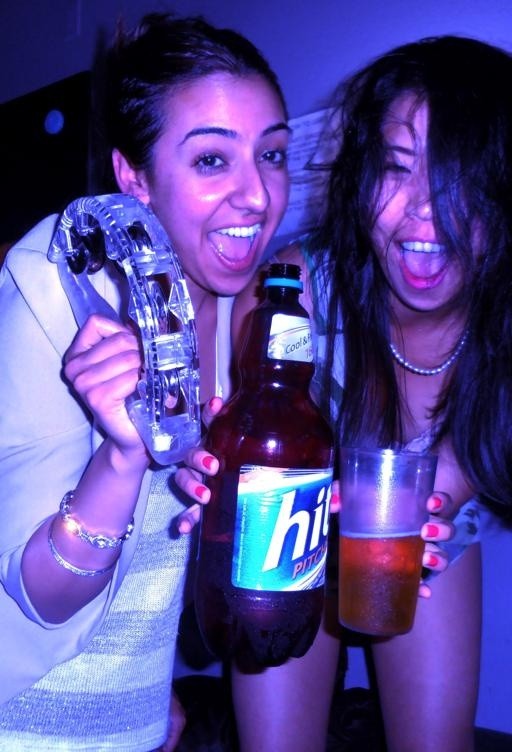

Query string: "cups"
[[338, 446, 438, 637]]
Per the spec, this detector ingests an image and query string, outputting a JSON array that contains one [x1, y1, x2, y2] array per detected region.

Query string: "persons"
[[173, 35, 512, 752], [0, 15, 291, 752]]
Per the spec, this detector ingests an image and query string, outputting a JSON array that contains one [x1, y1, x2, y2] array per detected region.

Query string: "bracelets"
[[59, 490, 135, 549], [47, 515, 118, 577]]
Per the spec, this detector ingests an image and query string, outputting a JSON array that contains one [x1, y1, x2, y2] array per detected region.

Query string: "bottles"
[[192, 262, 336, 667]]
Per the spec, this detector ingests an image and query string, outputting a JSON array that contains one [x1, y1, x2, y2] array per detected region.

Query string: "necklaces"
[[388, 330, 468, 375]]
